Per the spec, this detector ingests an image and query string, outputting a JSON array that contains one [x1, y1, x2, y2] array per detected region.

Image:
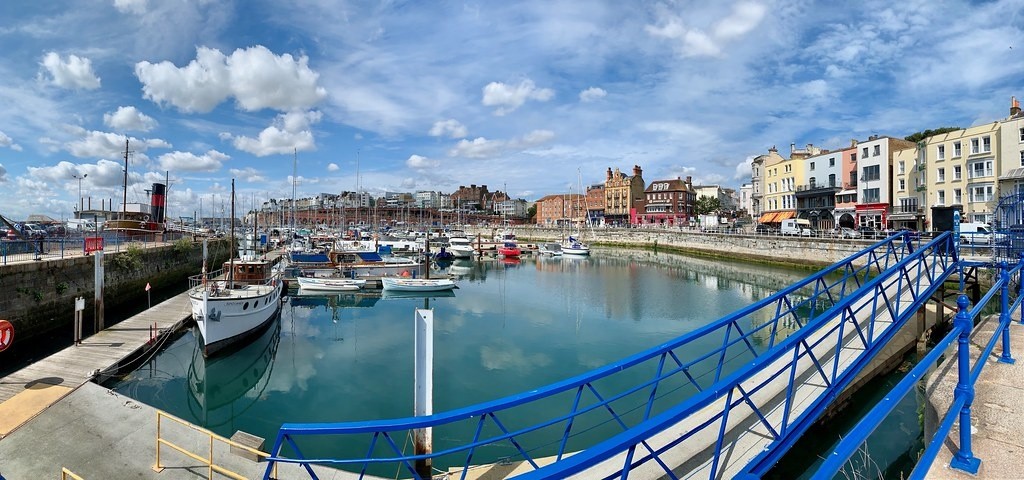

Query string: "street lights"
[[72, 174, 87, 236]]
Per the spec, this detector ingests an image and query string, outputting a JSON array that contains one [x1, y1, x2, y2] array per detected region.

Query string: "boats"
[[296, 276, 366, 291], [381, 276, 455, 292]]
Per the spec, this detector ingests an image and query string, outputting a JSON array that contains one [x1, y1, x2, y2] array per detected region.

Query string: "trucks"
[[781, 219, 811, 236]]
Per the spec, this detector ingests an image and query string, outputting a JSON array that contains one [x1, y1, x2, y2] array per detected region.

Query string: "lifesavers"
[[0, 327, 10, 349]]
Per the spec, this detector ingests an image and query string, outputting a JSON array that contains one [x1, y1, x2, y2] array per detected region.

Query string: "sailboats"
[[187, 178, 284, 355], [561, 166, 595, 255], [186, 302, 283, 430], [238, 148, 565, 279], [102, 139, 159, 242]]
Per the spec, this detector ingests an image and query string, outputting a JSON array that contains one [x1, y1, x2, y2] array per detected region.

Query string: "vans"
[[67, 218, 96, 232], [959, 222, 1007, 247], [23, 223, 47, 240]]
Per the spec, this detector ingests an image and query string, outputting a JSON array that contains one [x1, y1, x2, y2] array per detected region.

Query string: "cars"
[[841, 226, 922, 241]]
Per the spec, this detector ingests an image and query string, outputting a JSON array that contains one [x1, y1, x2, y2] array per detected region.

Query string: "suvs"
[[46, 225, 66, 238], [757, 224, 775, 234]]
